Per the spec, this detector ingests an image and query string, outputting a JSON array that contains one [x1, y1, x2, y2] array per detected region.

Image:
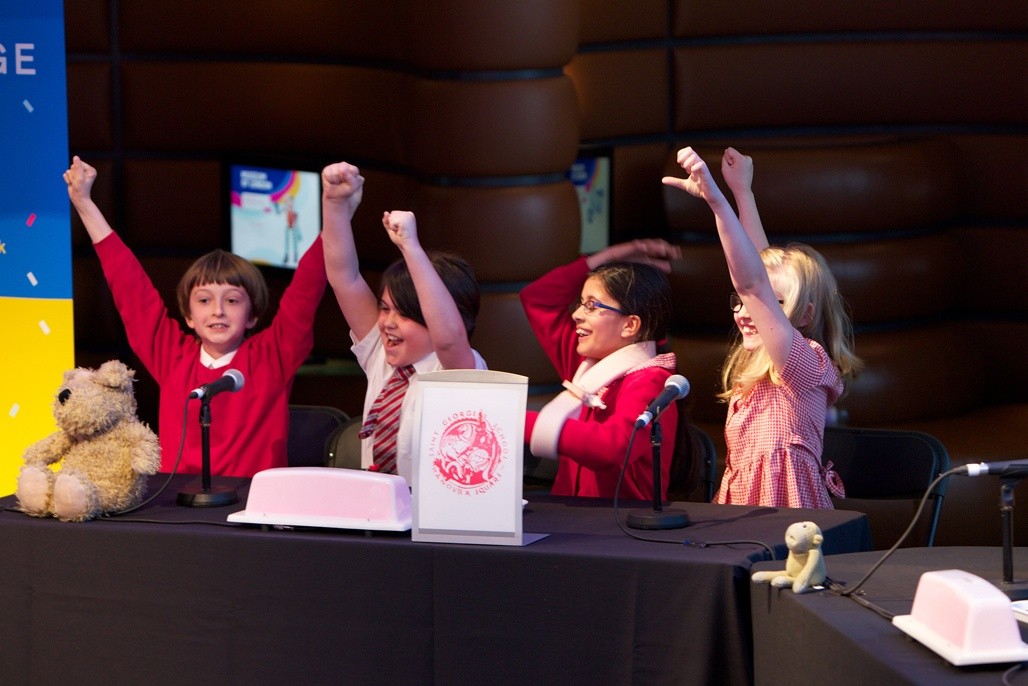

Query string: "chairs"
[[822, 426, 950, 548], [286, 405, 364, 469]]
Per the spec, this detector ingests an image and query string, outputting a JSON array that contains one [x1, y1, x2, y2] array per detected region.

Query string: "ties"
[[357, 363, 416, 475]]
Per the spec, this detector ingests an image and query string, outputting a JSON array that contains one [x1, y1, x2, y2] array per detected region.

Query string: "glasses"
[[729, 294, 784, 314], [575, 299, 624, 315]]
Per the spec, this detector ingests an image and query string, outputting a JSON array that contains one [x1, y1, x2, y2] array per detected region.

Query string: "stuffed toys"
[[18, 360, 161, 521], [751, 521, 827, 593]]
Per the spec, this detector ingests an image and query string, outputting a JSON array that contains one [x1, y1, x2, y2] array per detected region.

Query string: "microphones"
[[634, 375, 690, 429], [956, 459, 1028, 478], [188, 368, 245, 400]]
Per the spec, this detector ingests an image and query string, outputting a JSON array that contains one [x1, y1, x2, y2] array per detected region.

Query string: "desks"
[[0, 473, 869, 686], [750, 546, 1028, 686]]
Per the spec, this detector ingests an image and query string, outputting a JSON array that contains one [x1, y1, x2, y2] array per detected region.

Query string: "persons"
[[662, 146, 862, 510], [322, 162, 490, 480], [64, 155, 361, 478], [526, 239, 683, 501]]
